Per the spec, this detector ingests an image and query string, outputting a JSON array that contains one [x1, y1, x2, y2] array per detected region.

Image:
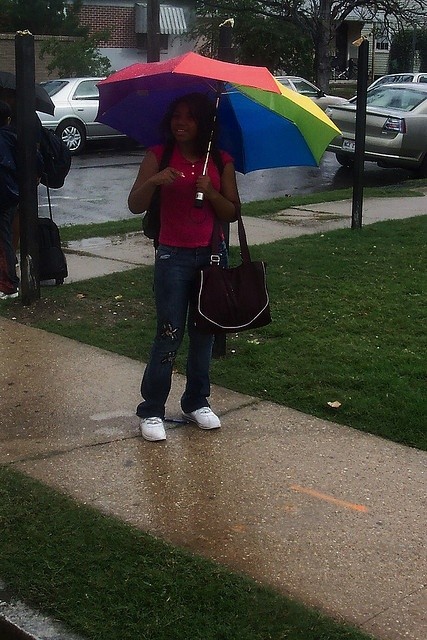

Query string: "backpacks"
[[40, 129, 72, 189], [142, 143, 223, 249]]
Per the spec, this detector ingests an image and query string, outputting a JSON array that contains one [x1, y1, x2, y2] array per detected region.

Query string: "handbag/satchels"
[[191, 260, 272, 334]]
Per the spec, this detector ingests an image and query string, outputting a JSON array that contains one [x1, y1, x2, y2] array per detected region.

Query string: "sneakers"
[[0, 291, 19, 300], [140, 416, 167, 442], [181, 407, 221, 430]]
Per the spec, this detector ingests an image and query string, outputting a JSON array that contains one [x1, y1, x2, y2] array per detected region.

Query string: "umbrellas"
[[93, 50, 344, 209], [0, 71, 55, 118]]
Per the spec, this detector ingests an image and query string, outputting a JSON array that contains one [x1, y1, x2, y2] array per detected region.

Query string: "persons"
[[0, 100, 24, 300], [127, 90, 241, 443], [10, 108, 42, 252]]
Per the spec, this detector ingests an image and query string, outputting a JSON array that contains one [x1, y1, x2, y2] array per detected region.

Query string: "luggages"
[[37, 172, 69, 285]]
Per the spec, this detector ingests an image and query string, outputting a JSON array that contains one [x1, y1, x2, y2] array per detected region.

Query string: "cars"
[[359, 72, 427, 83], [37, 78, 133, 154], [273, 75, 348, 111], [326, 83, 427, 170]]
